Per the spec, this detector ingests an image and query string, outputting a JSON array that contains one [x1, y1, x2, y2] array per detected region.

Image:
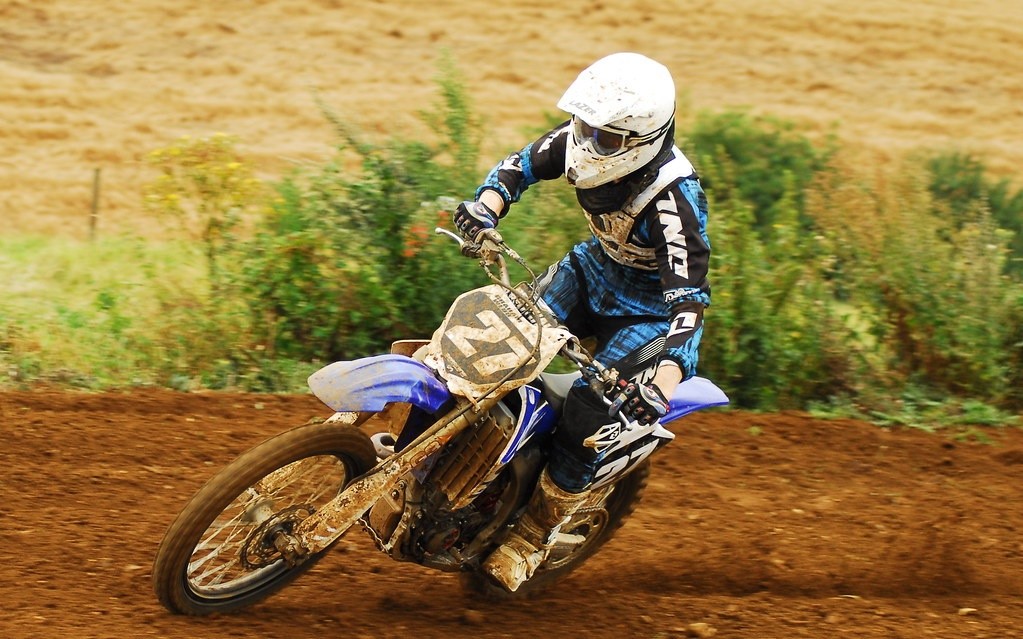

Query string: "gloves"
[[608, 383, 670, 426], [452, 200, 499, 241]]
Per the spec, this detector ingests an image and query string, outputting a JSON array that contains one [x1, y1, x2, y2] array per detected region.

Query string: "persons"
[[367, 53, 712, 592]]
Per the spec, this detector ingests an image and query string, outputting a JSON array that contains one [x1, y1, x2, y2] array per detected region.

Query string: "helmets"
[[555, 52, 677, 189]]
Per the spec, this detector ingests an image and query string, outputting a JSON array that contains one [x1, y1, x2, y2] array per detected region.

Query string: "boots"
[[481, 464, 591, 592]]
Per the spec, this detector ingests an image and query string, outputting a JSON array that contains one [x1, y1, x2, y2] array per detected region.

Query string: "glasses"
[[573, 113, 630, 157]]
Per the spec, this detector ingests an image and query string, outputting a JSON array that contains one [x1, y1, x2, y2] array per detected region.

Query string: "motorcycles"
[[149, 206, 729, 618]]
